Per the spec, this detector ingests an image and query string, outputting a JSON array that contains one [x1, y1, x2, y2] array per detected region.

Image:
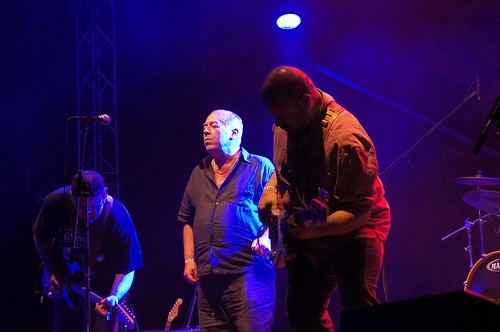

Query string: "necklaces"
[[214, 156, 237, 175]]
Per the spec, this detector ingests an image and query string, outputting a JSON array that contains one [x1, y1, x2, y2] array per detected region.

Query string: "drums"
[[464, 251, 500, 305]]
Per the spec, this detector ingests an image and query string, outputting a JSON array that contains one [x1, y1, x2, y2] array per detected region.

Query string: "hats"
[[72, 170, 104, 197]]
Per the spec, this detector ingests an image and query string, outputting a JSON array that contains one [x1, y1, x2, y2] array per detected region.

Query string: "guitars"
[[271, 184, 329, 269], [42, 253, 135, 330], [165, 298, 183, 330]]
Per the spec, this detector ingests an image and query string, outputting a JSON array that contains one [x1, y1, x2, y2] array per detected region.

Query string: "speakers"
[[339, 291, 500, 332]]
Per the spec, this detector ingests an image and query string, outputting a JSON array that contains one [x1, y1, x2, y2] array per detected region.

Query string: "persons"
[[258, 66, 391, 332], [178, 110, 276, 332], [33, 171, 143, 332]]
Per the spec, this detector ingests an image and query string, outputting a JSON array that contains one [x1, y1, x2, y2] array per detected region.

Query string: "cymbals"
[[451, 176, 500, 185], [461, 190, 500, 212]]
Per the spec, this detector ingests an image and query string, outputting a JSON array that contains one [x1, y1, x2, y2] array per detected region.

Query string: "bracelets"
[[185, 258, 195, 263], [263, 186, 277, 192], [112, 295, 118, 304]]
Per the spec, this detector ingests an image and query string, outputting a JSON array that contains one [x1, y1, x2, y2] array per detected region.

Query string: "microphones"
[[476, 78, 480, 101], [67, 113, 110, 125]]
